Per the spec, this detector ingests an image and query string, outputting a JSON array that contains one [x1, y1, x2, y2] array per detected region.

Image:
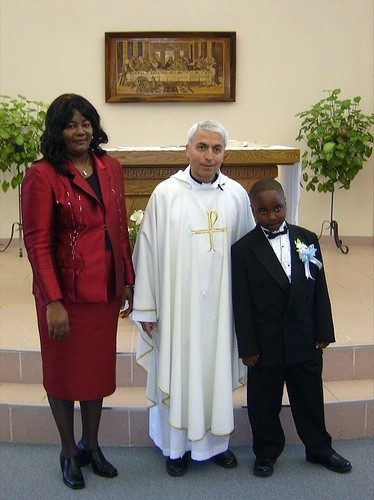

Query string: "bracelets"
[[127, 285, 134, 287]]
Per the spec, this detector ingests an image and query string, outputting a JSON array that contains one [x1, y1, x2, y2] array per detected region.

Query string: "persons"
[[231, 179, 352, 478], [132, 120, 256, 476], [21, 94, 135, 489]]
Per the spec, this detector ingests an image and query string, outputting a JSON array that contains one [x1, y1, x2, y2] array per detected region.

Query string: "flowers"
[[127, 209, 144, 249], [294, 238, 323, 279]]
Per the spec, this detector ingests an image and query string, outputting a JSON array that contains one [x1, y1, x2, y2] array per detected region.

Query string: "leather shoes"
[[306, 450, 351, 473], [254, 455, 277, 476]]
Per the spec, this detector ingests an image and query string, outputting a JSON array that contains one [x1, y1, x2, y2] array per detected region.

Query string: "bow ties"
[[263, 225, 288, 239]]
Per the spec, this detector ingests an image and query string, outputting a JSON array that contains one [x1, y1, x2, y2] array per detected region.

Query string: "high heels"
[[60, 451, 84, 489], [77, 441, 117, 477]]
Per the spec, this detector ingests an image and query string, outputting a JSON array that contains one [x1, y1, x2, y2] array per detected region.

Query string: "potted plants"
[[0, 94, 50, 193], [295, 89, 374, 193]]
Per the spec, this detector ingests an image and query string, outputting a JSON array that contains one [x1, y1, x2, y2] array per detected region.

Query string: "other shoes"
[[210, 449, 237, 468], [166, 452, 188, 476]]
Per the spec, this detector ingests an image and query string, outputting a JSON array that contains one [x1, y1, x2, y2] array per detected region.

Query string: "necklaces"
[[77, 157, 89, 176]]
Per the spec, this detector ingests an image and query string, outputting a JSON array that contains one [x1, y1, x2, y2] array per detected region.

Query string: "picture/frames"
[[104, 31, 237, 103]]
